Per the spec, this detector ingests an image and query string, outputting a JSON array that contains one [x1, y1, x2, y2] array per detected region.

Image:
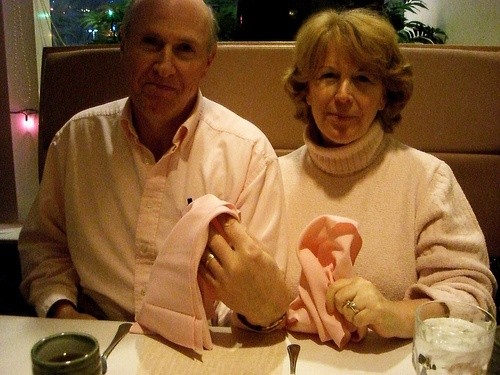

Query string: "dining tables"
[[0, 314, 416, 375]]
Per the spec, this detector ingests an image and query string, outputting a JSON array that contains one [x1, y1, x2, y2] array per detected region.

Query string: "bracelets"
[[262, 312, 287, 331]]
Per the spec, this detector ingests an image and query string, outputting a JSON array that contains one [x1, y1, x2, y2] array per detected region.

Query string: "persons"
[[16, 0, 289, 335], [277, 7, 497, 340]]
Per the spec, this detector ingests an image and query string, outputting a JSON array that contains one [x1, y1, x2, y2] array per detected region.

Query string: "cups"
[[30, 332, 102, 375], [412, 300, 497, 375]]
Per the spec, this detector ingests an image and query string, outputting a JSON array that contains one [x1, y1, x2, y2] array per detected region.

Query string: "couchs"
[[38, 42, 500, 323]]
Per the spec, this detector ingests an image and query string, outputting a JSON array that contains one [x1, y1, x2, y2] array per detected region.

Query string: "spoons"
[[100, 323, 133, 374]]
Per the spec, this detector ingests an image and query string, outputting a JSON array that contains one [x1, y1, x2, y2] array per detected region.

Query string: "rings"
[[347, 300, 360, 313], [205, 254, 215, 267]]
